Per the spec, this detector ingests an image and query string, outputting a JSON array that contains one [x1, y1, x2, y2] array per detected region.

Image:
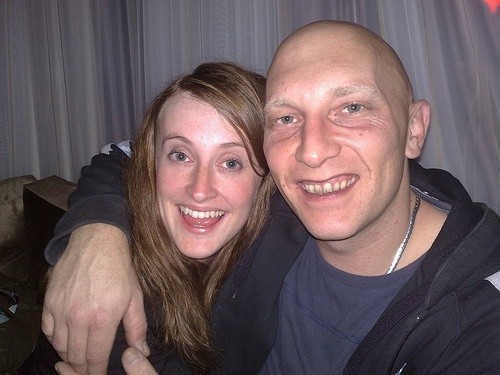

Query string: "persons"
[[0, 61, 269, 375], [15, 19, 499, 375]]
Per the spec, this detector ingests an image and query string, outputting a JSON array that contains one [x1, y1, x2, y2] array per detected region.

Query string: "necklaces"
[[383, 192, 423, 274]]
